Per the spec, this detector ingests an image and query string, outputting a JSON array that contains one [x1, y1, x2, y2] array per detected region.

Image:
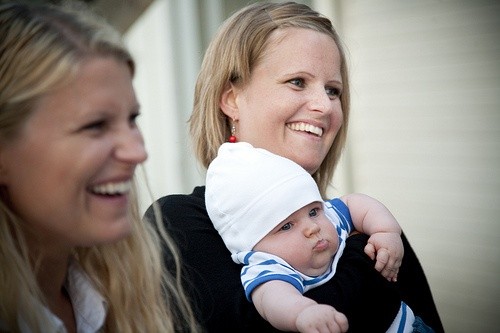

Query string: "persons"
[[141, 1, 446, 333], [0, 0, 199, 333], [205, 142, 437, 333]]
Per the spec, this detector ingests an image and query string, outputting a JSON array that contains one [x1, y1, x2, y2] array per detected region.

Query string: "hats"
[[205, 142, 325, 254]]
